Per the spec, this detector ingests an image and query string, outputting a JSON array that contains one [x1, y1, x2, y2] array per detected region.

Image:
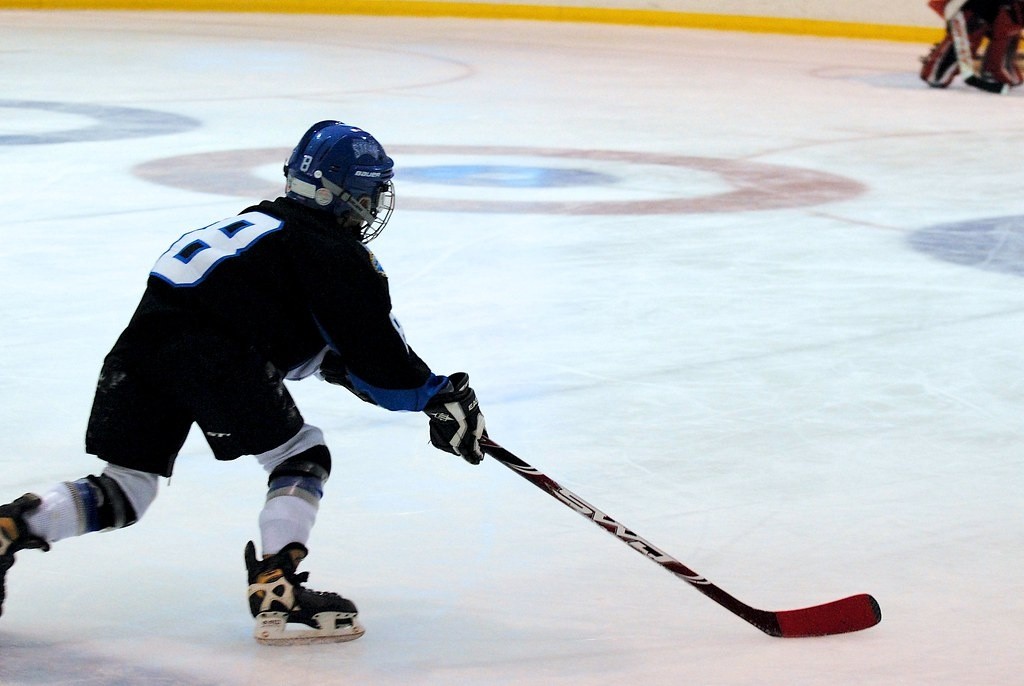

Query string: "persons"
[[0, 120, 489, 646], [920, 0, 1024, 94]]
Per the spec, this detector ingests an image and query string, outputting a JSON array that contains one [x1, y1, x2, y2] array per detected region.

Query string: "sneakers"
[[246, 540, 360, 636], [0, 490, 52, 617]]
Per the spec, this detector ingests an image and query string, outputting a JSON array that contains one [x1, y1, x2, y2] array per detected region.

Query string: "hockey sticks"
[[944, 9, 1011, 98], [480, 434, 882, 640]]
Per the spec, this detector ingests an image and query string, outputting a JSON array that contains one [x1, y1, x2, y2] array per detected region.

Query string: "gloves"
[[428, 374, 487, 464]]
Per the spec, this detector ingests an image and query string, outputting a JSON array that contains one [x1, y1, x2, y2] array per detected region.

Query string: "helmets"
[[284, 119, 393, 211]]
